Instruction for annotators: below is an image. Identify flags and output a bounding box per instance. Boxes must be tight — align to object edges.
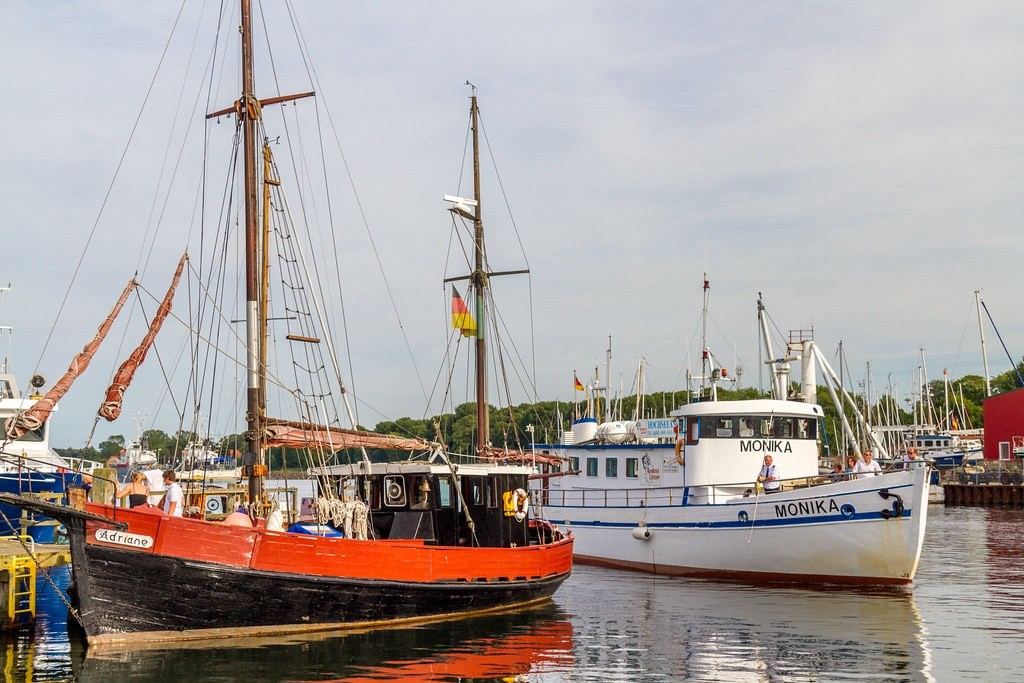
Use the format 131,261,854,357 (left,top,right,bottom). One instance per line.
452,285,477,337
575,375,584,391
951,416,957,430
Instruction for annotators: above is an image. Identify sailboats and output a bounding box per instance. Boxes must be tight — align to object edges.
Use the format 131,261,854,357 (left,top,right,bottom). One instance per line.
818,340,984,485
59,0,575,649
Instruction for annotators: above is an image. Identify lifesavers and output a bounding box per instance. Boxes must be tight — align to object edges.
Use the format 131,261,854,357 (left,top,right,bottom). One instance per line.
513,488,529,519
1010,473,1023,485
502,492,516,516
1001,473,1011,485
675,439,684,465
210,464,218,471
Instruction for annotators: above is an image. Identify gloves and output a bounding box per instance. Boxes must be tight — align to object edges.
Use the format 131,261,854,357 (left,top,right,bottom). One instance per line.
756,485,761,495
757,476,764,483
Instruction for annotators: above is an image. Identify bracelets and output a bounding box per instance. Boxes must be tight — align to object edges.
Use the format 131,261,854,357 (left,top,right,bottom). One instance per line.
116,489,120,490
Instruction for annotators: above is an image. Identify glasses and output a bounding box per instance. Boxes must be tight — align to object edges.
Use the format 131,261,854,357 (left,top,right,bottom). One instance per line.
908,452,915,455
833,467,836,469
863,454,868,456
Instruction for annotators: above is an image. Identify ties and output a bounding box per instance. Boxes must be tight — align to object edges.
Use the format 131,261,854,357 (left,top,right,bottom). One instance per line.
766,468,769,478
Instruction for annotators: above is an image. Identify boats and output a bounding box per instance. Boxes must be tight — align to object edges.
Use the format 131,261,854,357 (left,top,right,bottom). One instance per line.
104,440,200,494
162,440,245,484
0,283,103,546
515,272,934,587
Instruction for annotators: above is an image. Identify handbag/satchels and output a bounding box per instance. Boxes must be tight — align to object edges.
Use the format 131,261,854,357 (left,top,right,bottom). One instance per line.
157,495,165,510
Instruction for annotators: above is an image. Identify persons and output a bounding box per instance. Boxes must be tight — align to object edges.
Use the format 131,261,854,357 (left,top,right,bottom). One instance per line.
817,450,883,484
115,471,150,508
79,472,92,502
755,456,780,496
162,470,185,517
904,448,926,470
